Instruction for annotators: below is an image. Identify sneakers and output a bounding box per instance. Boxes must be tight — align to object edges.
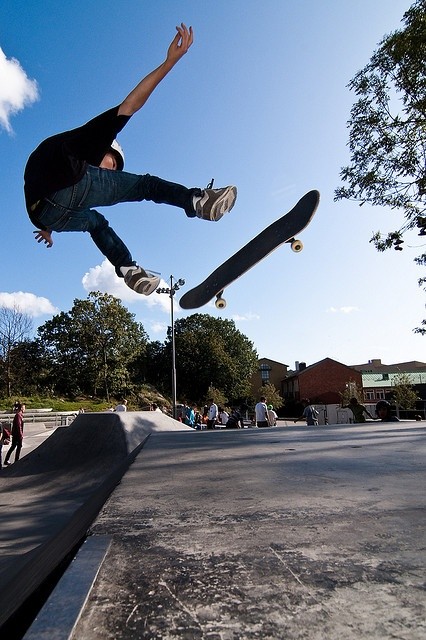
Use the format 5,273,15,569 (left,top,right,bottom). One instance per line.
195,178,237,221
123,267,161,295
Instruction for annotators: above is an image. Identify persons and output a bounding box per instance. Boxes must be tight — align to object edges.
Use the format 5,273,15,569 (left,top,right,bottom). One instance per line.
25,23,237,295
341,398,379,423
375,401,400,422
152,398,244,430
293,398,320,426
114,399,127,412
254,397,270,428
267,404,278,427
0,418,4,470
414,414,422,421
4,402,25,465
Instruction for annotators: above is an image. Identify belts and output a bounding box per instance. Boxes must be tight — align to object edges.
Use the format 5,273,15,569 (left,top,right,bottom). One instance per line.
31,195,50,216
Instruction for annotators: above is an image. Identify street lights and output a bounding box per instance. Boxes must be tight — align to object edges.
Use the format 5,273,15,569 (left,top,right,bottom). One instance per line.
156,275,185,419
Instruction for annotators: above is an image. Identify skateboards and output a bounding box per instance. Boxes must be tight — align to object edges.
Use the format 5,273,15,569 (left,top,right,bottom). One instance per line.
179,190,320,309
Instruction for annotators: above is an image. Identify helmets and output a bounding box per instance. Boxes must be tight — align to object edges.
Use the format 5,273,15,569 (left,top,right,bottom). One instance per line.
376,400,391,410
109,138,124,171
